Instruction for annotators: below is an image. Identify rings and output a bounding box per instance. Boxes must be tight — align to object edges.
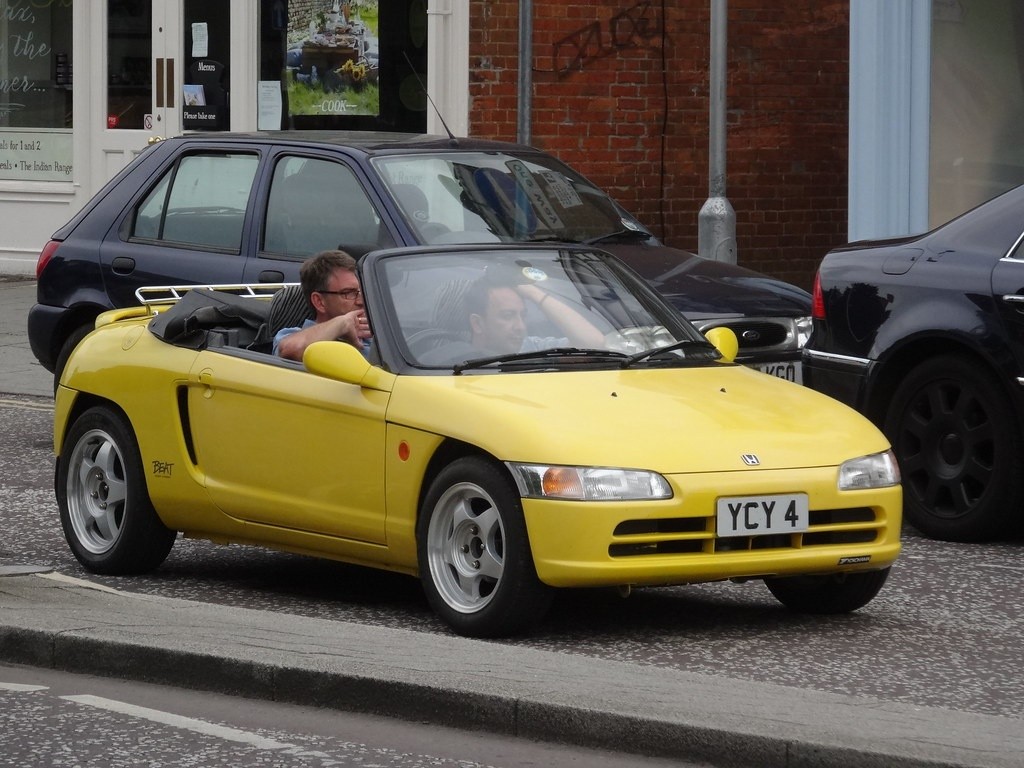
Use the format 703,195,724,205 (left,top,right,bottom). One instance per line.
359,317,362,323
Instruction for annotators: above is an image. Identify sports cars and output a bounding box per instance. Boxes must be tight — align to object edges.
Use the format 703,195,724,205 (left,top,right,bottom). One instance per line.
50,240,904,638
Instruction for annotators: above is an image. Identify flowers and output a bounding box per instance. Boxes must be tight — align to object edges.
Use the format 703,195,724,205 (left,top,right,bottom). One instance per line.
333,58,365,81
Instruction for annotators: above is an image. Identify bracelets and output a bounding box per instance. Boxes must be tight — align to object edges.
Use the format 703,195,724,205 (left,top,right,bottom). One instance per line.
538,293,549,308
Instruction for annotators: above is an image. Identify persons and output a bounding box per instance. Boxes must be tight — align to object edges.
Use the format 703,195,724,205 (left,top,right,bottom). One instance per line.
272,251,374,367
440,275,607,366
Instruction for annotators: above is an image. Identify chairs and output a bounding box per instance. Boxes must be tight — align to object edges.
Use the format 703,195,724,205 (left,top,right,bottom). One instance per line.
429,274,474,346
281,168,450,255
266,285,313,337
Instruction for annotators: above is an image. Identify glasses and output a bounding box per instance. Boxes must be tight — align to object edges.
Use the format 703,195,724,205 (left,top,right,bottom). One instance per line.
319,290,362,300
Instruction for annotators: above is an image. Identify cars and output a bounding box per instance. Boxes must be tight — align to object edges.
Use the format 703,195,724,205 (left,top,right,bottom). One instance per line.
808,180,1024,542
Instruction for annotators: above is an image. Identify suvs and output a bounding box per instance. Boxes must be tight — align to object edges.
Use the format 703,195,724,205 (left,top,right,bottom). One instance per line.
24,125,815,395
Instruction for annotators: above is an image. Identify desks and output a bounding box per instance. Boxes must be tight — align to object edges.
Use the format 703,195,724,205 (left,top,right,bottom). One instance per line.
302,43,364,74
